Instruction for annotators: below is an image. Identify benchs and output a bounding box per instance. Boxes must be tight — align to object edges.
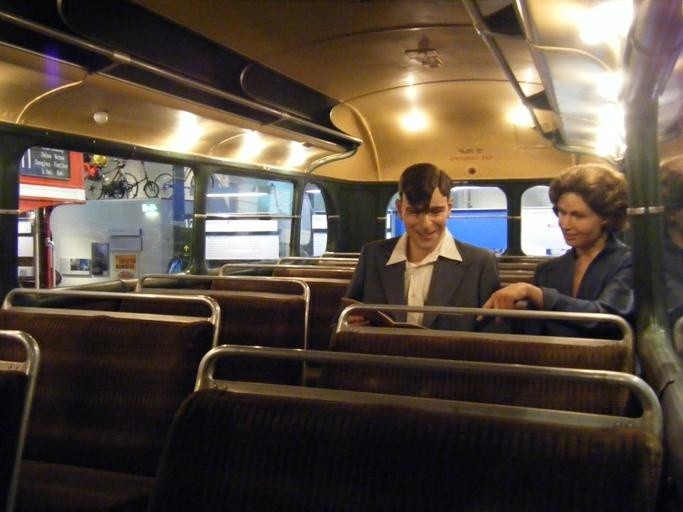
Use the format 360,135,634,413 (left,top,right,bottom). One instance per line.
1,252,666,511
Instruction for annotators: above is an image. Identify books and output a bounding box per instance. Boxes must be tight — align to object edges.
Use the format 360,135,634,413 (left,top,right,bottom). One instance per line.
339,296,431,331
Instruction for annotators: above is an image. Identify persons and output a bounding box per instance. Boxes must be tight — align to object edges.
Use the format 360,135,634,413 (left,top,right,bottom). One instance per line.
329,163,500,338
477,163,637,340
657,166,683,332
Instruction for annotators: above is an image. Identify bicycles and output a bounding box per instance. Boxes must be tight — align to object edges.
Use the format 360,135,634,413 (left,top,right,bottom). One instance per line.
85,155,215,200
